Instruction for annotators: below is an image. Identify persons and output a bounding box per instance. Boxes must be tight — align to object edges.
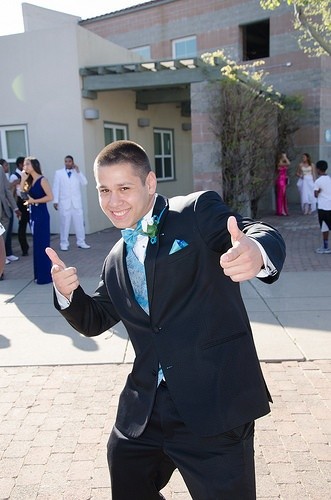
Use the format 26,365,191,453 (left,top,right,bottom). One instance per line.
10,156,36,256
46,141,286,500
296,153,317,215
276,148,290,216
0,158,21,281
312,160,331,254
52,155,92,250
20,156,54,285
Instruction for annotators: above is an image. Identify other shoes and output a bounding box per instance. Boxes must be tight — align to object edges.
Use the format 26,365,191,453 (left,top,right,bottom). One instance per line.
6,255,18,261
22,251,29,256
61,246,68,251
77,243,90,249
5,260,10,264
316,247,331,253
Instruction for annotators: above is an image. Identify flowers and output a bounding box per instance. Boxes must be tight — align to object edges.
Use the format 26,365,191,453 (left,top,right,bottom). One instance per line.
140,215,158,238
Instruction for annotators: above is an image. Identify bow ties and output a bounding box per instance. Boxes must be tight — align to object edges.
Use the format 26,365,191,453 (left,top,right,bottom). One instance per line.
67,169,73,173
121,218,150,249
